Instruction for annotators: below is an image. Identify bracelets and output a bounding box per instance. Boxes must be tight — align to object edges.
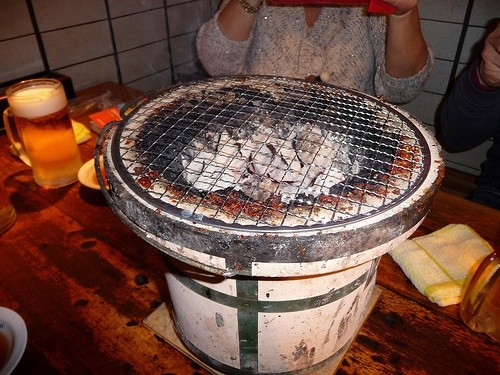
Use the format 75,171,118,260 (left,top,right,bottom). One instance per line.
238,0,260,14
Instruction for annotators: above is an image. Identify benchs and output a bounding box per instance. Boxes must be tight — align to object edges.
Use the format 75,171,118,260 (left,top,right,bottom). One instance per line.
439,159,479,199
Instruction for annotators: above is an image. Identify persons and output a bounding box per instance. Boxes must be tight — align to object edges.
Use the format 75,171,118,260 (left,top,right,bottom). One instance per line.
197,0,435,106
432,22,500,209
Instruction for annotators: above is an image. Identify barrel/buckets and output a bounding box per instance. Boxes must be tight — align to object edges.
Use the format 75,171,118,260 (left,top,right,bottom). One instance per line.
95,75,445,375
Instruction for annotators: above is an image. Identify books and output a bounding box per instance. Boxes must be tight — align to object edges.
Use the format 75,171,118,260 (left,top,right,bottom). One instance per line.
88,96,147,139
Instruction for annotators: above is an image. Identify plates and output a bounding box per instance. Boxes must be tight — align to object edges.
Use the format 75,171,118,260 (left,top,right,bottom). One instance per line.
0,305,27,375
77,153,109,191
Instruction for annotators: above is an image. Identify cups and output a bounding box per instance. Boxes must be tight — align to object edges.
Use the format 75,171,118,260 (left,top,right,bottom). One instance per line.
4,78,82,188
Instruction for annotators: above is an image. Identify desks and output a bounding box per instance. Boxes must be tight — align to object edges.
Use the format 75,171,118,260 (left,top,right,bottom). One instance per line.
0,80,500,375
